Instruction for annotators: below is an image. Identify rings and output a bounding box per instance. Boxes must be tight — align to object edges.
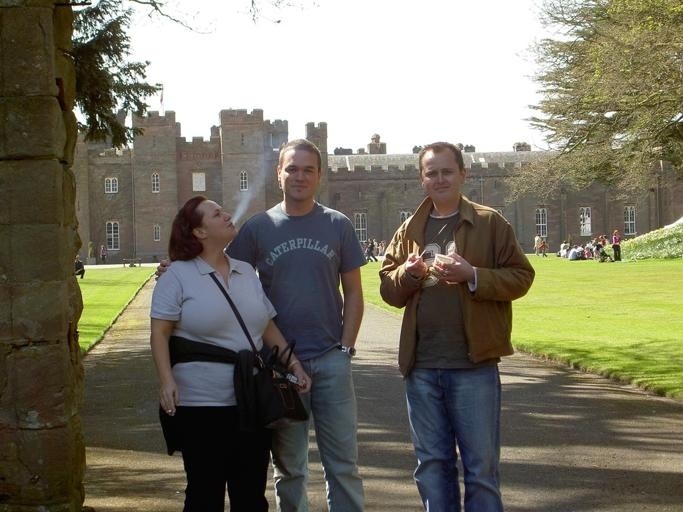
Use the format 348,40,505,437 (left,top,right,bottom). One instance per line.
443,271,449,277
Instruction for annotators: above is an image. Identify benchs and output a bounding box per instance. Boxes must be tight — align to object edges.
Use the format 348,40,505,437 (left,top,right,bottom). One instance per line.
122,258,140,267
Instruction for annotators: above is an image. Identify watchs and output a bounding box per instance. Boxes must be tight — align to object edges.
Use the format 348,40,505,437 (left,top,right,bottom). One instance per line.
339,346,358,356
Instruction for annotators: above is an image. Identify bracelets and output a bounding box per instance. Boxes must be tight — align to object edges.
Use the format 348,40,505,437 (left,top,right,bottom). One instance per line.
287,359,300,372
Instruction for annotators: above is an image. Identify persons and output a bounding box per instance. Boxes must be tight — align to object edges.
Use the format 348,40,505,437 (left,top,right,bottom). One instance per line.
376,139,537,510
99,246,107,265
148,193,313,512
154,136,370,511
360,237,387,264
73,255,86,279
532,228,624,264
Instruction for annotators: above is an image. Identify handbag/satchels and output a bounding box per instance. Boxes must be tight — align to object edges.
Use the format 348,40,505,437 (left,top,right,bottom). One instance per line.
234,349,298,427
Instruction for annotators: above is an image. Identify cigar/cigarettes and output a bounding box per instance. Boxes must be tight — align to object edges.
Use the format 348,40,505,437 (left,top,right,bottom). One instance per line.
166,409,172,413
